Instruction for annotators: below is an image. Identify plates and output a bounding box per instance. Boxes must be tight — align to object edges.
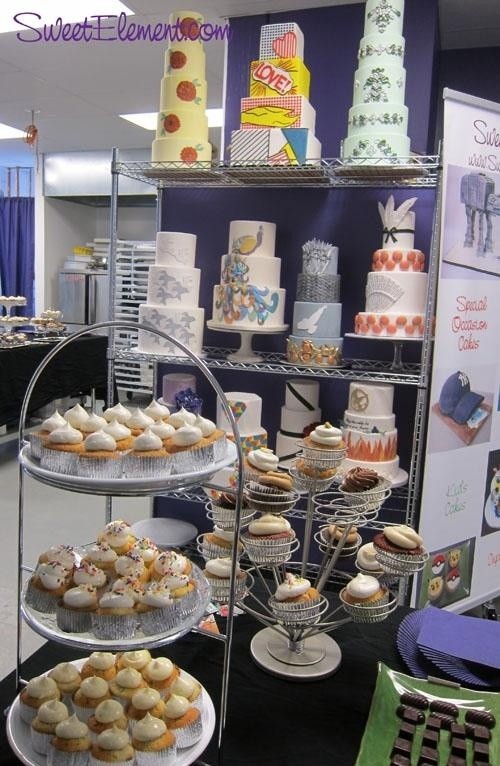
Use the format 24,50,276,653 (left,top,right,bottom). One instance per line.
34,326,64,331
484,494,500,531
34,336,68,343
349,660,499,765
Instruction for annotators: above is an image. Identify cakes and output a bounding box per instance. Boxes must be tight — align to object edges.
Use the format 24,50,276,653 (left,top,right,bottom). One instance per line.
343,0,411,165
355,195,429,338
0,296,67,341
137,230,205,357
231,23,323,167
213,219,287,327
152,10,212,169
286,237,345,369
428,550,461,601
489,467,500,517
19,373,424,766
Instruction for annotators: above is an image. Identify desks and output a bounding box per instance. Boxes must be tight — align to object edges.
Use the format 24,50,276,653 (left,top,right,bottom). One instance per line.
0,552,500,766
0,325,108,435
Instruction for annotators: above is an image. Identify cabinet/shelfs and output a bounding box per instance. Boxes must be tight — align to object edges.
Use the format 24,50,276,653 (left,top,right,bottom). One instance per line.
105,138,444,606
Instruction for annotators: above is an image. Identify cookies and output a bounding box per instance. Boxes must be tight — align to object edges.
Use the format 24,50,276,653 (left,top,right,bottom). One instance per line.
389,693,496,766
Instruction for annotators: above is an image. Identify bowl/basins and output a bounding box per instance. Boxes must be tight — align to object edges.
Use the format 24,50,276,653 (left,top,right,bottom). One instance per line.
89,255,107,266
